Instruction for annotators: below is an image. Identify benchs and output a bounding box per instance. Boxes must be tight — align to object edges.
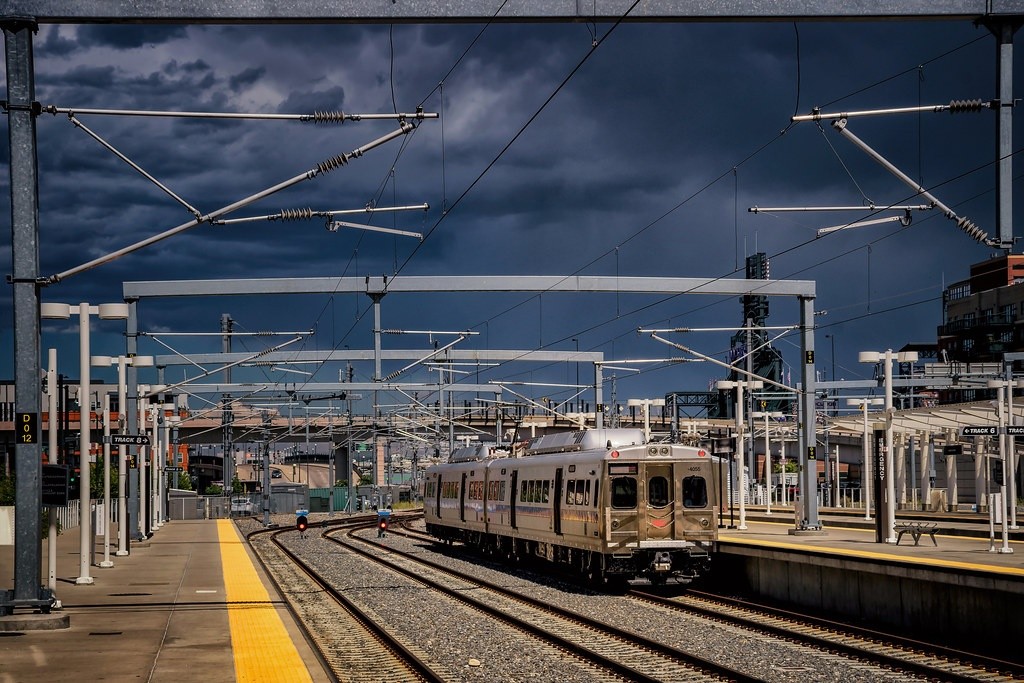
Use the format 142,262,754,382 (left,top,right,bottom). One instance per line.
894,521,941,546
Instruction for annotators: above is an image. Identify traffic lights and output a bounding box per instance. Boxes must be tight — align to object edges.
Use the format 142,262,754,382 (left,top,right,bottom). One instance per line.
377,516,388,532
296,516,308,531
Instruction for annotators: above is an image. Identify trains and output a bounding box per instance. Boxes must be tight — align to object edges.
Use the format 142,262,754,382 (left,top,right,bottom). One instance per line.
423,428,721,596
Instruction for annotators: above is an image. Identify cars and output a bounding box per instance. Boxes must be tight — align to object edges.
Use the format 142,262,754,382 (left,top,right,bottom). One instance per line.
230,497,258,517
360,489,393,513
271,469,282,479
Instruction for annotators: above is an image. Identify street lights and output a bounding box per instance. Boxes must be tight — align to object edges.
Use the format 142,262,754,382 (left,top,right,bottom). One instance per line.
147,415,180,528
987,379,1024,530
125,385,168,540
716,380,764,529
91,356,154,556
826,334,835,396
39,303,127,586
858,350,918,543
137,402,176,530
572,339,579,413
628,398,666,442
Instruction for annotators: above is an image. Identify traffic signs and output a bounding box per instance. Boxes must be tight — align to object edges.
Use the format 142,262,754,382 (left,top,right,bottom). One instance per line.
111,434,152,447
